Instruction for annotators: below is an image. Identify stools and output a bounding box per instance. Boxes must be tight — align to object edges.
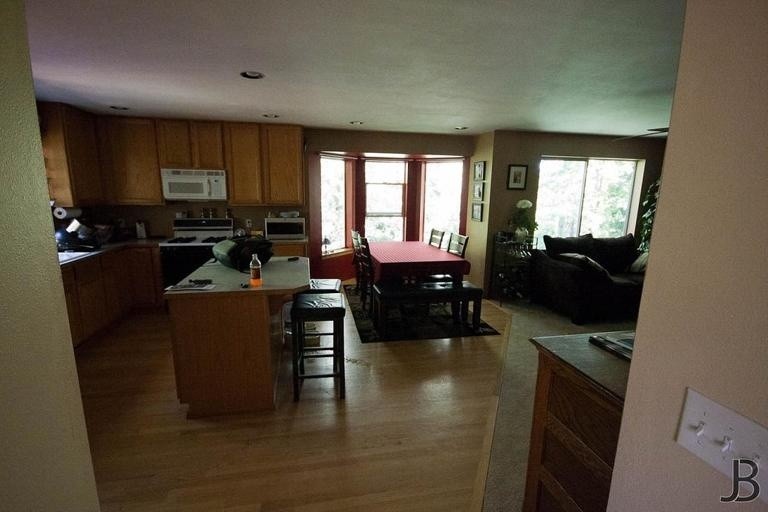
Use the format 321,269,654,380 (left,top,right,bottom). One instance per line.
291,293,346,402
293,278,341,352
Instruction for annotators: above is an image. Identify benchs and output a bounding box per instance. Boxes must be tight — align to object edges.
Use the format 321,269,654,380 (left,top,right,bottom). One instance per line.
373,281,482,330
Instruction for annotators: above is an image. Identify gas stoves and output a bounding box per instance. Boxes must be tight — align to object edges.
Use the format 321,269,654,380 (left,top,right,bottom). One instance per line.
158,236,229,245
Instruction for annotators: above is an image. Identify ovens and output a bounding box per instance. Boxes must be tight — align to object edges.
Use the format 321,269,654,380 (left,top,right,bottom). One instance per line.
160,246,216,303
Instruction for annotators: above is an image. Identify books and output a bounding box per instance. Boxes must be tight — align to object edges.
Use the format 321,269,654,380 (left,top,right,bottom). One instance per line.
589,333,636,362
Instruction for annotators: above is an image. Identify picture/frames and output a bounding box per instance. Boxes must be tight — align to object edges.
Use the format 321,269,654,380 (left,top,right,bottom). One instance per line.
471,203,483,222
474,161,485,181
507,164,528,190
472,183,484,201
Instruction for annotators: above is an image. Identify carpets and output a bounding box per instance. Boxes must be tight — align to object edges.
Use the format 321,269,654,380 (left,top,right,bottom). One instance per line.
471,300,636,512
342,277,501,343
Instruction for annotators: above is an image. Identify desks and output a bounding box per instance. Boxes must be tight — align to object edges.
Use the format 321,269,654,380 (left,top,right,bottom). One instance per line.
522,331,633,512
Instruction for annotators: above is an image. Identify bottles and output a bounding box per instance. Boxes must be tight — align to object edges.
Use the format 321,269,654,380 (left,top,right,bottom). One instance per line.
249,253,261,288
200,207,229,218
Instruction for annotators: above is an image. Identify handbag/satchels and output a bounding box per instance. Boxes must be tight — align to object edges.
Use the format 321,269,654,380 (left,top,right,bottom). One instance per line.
212,235,274,272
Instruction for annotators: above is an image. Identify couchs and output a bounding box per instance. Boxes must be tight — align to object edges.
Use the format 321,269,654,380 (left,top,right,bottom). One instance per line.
534,250,646,323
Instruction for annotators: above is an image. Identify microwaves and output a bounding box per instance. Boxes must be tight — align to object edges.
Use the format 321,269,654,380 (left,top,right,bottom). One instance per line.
160,168,229,202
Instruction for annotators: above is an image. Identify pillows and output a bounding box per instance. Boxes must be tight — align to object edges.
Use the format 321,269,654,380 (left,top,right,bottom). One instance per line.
626,252,649,273
542,234,594,260
593,232,636,273
560,253,613,282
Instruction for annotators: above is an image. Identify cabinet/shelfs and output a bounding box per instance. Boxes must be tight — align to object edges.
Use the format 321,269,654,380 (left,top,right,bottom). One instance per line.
61,246,165,348
272,245,305,257
96,116,172,206
223,122,306,207
491,232,538,307
154,118,223,170
36,102,104,208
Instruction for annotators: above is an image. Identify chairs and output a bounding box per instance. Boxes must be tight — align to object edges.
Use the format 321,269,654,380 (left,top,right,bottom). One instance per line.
351,230,372,312
408,229,469,315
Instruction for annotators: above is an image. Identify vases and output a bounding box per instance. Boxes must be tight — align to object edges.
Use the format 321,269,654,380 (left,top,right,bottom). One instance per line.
516,227,529,241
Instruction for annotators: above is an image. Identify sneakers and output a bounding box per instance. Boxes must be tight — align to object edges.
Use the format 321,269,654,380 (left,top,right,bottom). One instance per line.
283,323,320,347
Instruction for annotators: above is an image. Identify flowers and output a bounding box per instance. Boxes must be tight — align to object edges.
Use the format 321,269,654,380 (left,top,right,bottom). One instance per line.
508,200,539,231
